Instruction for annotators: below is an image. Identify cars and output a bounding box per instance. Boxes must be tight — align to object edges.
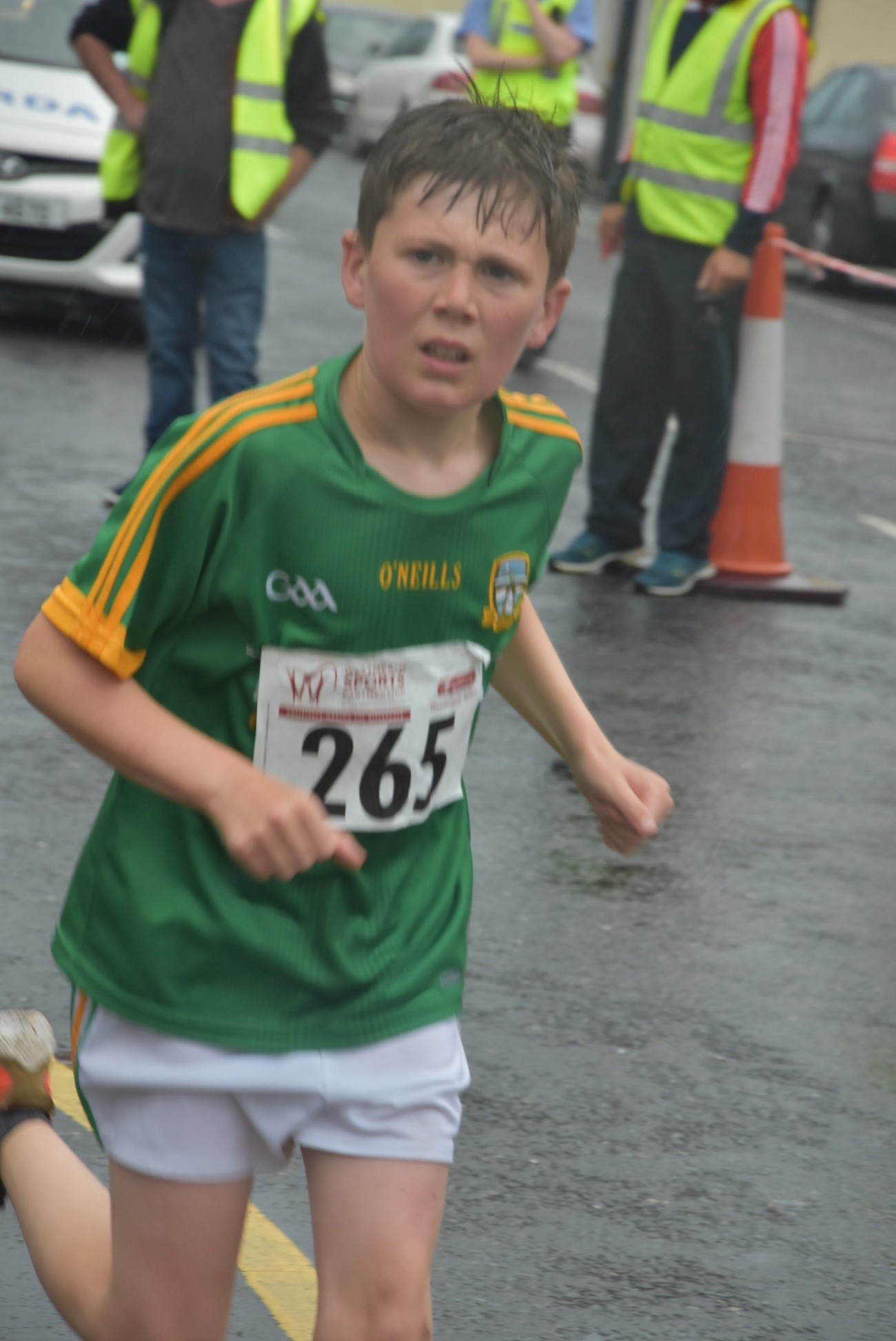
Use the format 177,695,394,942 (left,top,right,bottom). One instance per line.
344,16,609,174
0,0,210,339
763,64,895,292
316,2,388,116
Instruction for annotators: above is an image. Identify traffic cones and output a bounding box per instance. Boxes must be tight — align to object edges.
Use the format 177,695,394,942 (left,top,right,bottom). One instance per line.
604,221,856,607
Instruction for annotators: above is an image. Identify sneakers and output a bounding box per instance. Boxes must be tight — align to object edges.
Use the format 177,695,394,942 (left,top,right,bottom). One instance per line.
636,548,718,598
547,527,647,574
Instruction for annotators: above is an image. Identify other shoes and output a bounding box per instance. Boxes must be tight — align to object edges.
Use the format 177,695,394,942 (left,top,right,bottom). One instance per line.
103,482,130,505
1,1007,58,1112
516,351,535,367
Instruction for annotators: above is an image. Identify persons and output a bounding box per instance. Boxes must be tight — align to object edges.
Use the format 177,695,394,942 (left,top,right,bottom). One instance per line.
546,1,809,601
460,2,600,369
0,100,669,1339
68,0,335,503
